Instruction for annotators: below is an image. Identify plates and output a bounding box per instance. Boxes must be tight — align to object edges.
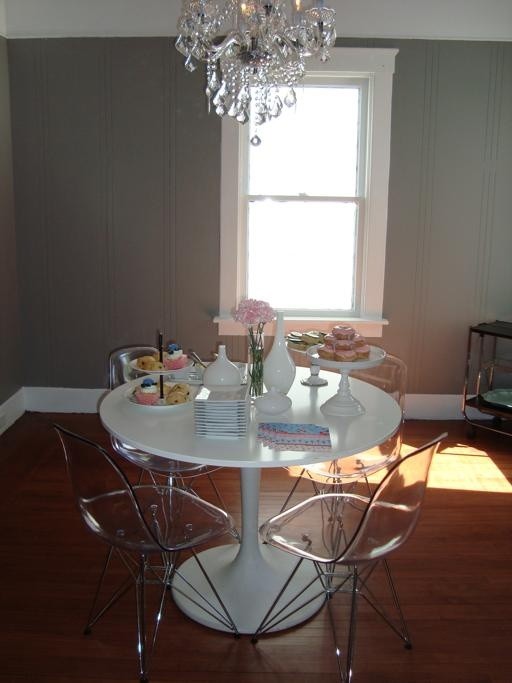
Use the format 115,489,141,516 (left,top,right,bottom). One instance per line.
192,383,252,441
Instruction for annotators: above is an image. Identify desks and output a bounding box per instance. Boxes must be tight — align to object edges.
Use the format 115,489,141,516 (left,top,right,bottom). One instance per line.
99,361,403,637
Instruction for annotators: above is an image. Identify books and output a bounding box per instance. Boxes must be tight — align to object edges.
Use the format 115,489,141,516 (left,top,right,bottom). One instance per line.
194,384,252,439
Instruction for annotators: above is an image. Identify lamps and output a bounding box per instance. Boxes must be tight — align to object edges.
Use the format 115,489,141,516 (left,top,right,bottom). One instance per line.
174,1,337,148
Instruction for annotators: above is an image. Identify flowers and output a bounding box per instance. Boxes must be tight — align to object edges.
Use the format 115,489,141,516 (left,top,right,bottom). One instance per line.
231,298,276,397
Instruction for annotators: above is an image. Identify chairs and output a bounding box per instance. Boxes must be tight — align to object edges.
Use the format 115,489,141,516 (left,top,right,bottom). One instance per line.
54,425,241,683
251,431,447,683
108,345,241,540
277,355,409,516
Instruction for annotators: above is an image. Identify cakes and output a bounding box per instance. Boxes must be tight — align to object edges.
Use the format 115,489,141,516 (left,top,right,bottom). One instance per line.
134,343,192,405
287,325,371,362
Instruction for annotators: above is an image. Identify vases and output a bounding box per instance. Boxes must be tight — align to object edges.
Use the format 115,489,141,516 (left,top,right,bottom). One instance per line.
245,324,264,400
